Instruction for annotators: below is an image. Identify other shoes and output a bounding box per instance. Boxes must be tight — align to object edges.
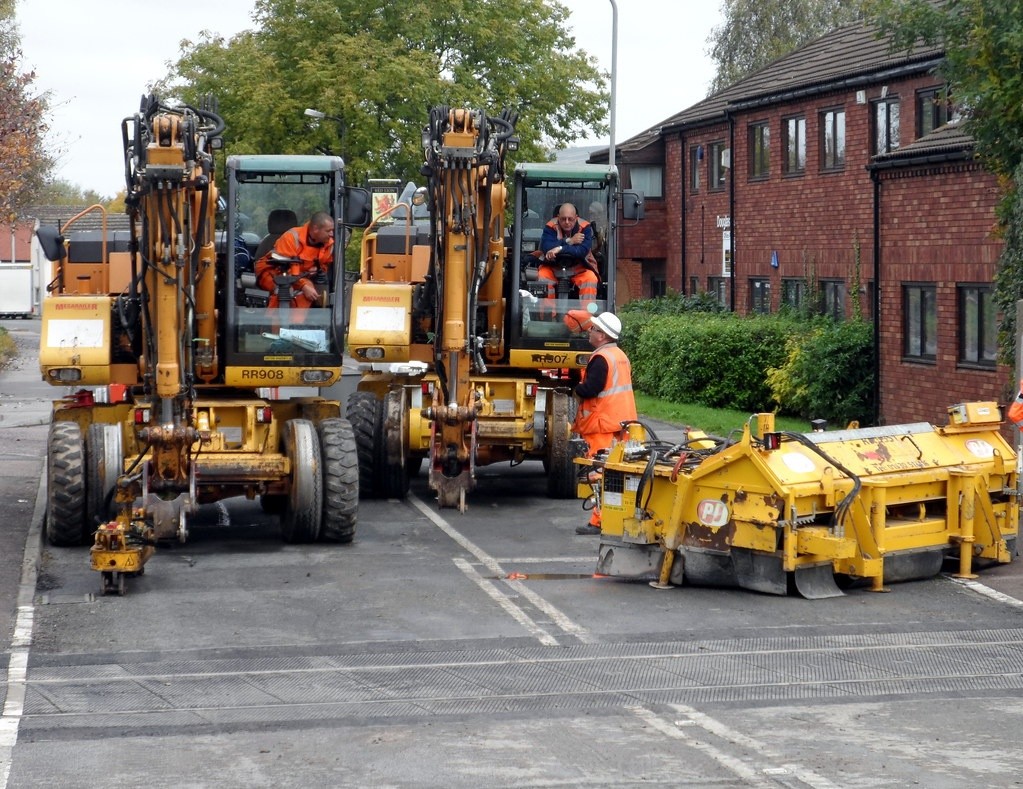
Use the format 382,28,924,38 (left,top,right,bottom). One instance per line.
575,524,602,535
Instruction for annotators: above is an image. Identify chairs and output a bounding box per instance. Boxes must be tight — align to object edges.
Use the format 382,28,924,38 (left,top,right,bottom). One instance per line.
257,207,302,263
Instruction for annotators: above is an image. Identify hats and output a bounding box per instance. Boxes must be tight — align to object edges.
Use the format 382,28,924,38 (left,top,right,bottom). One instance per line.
590,311,622,341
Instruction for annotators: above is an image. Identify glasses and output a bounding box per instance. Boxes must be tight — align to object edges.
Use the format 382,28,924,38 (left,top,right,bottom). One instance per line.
558,216,577,222
591,325,604,334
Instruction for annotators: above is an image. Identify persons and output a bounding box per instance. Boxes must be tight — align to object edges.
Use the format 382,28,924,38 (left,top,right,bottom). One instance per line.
566,312,642,535
535,204,597,318
254,212,336,337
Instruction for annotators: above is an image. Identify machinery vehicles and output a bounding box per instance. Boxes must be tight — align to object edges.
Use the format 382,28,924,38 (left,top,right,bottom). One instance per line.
346,93,626,507
32,85,363,599
